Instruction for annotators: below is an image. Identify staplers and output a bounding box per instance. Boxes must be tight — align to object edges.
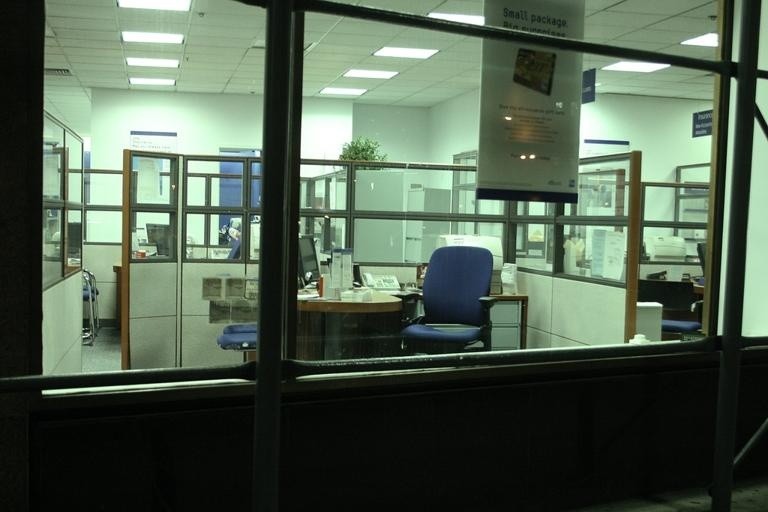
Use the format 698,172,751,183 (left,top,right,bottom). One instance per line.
646,270,666,281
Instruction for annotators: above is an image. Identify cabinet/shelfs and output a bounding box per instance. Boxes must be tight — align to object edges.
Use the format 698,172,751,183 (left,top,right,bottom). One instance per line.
415,295,529,351
638,279,694,319
636,301,664,341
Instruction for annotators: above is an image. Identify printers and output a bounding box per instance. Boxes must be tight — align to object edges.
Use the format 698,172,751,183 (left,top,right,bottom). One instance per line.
644,235,687,262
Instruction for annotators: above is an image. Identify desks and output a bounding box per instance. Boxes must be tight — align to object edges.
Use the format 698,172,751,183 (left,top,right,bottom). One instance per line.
298,288,403,361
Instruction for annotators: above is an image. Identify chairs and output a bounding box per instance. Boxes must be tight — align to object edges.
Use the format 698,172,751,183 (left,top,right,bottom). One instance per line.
662,319,701,341
393,246,495,355
216,323,258,350
83,268,102,345
227,243,238,258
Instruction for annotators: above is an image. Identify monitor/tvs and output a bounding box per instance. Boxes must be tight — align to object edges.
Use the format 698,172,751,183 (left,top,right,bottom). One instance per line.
146,223,175,255
293,237,321,285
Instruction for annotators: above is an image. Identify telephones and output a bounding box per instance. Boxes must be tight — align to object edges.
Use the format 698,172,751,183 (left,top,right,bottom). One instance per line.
361,272,401,291
